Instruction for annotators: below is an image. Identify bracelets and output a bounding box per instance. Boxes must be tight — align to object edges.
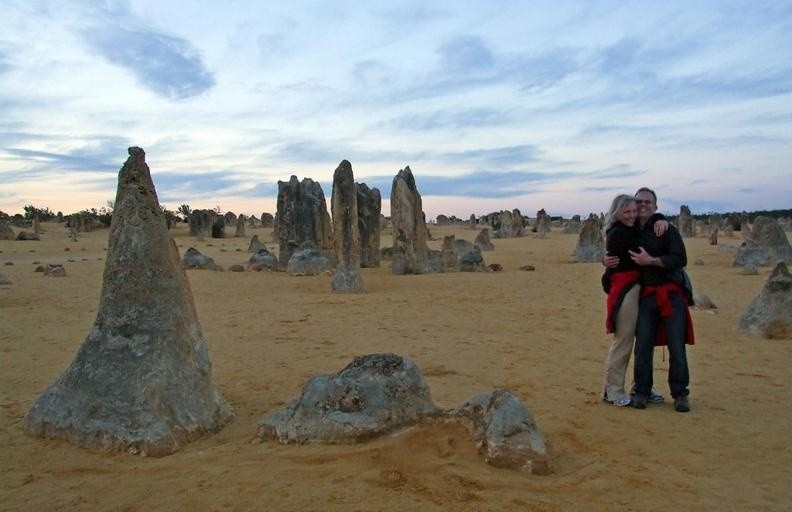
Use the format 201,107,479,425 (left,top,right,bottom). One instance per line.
649,257,656,265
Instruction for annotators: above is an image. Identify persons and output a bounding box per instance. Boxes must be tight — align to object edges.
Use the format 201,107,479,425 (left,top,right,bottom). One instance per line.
599,187,692,412
596,194,668,406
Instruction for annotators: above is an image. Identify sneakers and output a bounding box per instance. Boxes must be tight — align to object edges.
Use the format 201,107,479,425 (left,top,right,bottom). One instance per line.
631,386,664,403
604,392,631,406
633,391,648,408
674,396,690,411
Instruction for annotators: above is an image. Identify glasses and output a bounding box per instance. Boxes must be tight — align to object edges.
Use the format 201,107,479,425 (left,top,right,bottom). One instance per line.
636,198,651,204
